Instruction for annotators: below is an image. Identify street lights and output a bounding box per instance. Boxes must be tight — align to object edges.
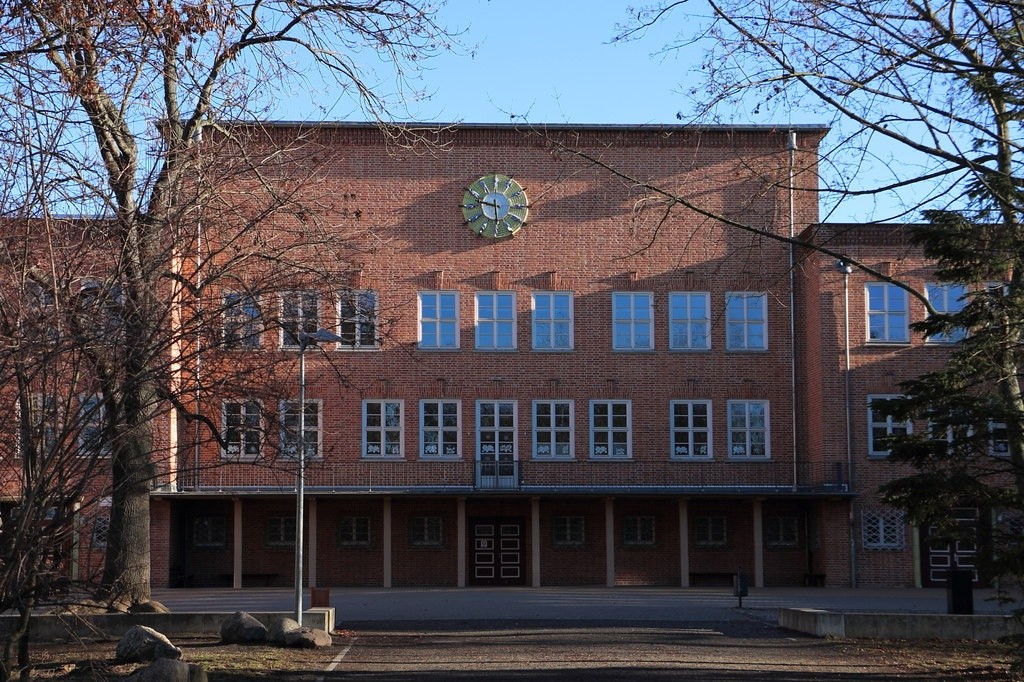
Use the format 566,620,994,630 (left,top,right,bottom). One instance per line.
295,329,354,625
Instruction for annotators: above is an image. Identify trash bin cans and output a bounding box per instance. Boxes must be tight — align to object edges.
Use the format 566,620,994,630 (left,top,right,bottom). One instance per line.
946,568,973,615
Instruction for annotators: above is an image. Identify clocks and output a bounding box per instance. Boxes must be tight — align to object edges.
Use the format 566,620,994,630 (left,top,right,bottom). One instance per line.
457,172,532,241
